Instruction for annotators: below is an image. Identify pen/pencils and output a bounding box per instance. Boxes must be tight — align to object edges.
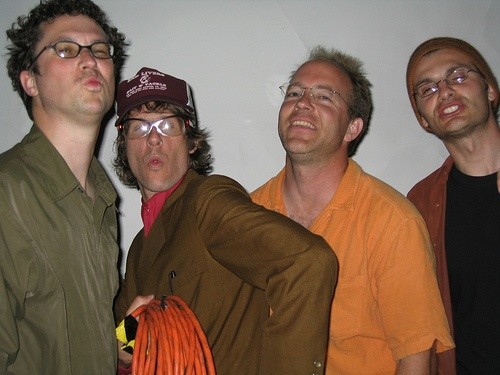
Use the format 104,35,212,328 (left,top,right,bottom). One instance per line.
168,269,177,294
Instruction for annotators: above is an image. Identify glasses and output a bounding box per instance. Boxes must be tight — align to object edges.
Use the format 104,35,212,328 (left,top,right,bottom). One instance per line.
277,80,356,112
30,41,117,68
407,67,490,100
112,115,190,138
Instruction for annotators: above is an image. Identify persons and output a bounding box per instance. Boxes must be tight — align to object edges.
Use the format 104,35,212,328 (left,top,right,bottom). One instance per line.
0,0,130,375
112,67,338,375
406,37,500,375
248,44,456,375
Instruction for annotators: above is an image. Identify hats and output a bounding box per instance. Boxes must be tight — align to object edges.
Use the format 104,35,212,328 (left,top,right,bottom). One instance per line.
114,67,194,128
